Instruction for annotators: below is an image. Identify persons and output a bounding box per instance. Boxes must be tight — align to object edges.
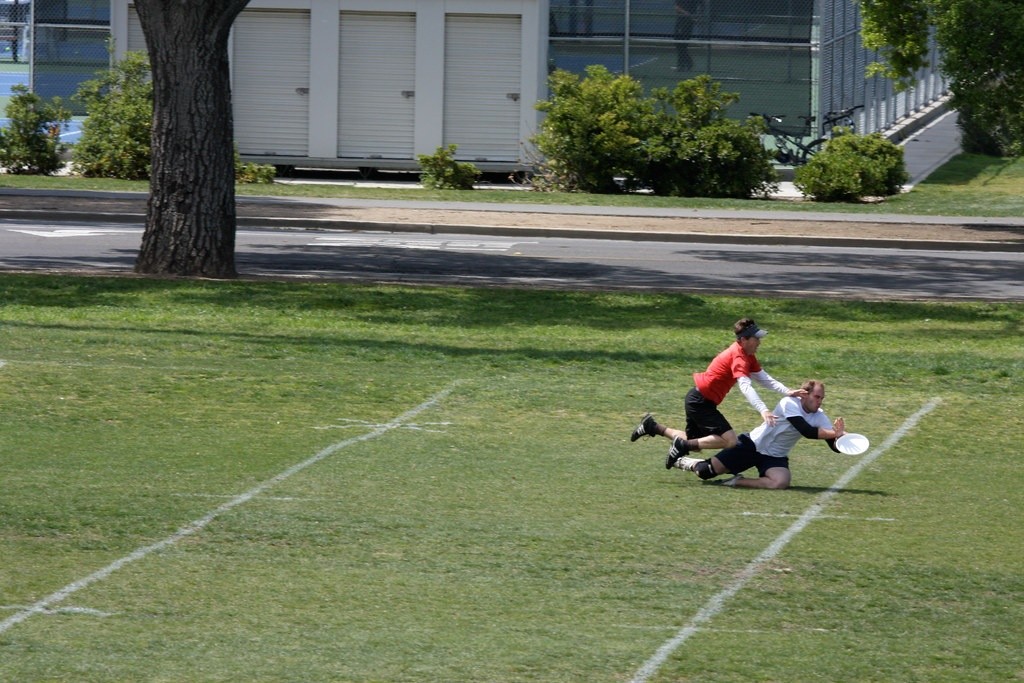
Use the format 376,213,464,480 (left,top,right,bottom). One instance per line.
631,317,808,469
672,378,851,491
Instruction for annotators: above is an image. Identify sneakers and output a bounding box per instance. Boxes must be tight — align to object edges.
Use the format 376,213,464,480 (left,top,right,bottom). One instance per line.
674,457,705,471
631,414,657,442
720,474,744,487
666,436,689,470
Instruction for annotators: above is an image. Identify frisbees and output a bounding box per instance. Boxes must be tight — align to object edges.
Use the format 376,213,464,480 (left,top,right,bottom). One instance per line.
835,432,870,456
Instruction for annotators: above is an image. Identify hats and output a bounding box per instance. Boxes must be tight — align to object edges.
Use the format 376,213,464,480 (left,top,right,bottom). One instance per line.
736,324,768,338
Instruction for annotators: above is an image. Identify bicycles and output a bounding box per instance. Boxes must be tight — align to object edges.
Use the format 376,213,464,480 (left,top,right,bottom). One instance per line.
747,102,864,166
